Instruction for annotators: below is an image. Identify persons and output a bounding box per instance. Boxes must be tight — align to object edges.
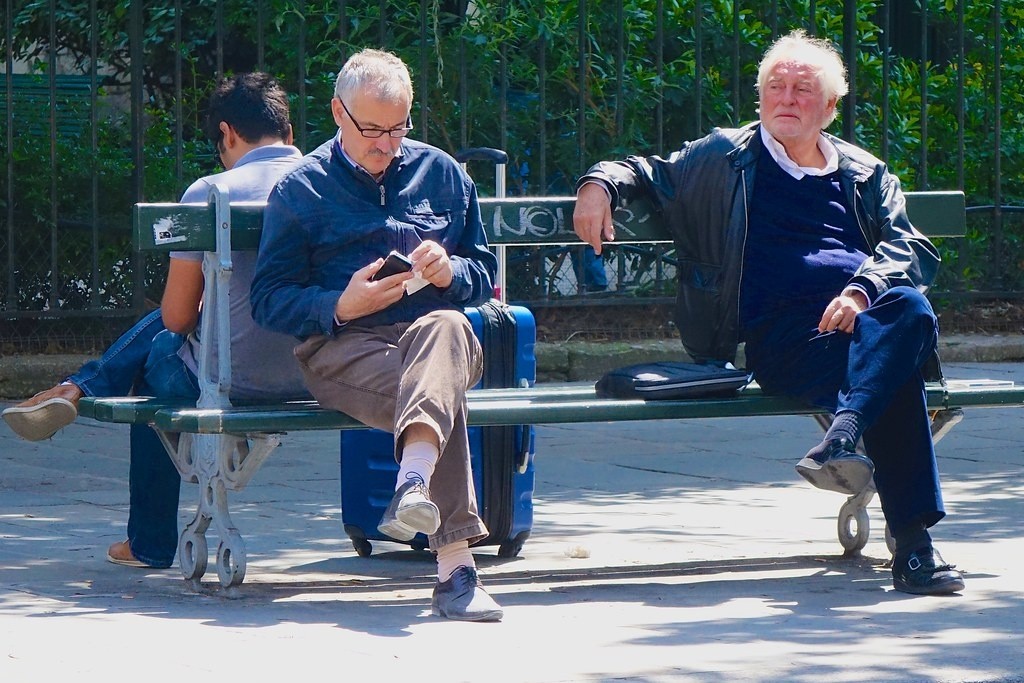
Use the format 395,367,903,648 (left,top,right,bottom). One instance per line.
248,49,504,621
1,71,302,567
572,32,965,594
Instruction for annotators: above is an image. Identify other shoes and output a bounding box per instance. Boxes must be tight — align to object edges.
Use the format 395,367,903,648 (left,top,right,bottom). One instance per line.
106,538,150,567
2,383,84,442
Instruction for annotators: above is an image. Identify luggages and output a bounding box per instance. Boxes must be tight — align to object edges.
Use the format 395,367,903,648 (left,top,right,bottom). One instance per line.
340,148,538,560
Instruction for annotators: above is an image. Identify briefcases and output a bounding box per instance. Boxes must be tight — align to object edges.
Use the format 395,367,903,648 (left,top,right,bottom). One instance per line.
594,362,753,400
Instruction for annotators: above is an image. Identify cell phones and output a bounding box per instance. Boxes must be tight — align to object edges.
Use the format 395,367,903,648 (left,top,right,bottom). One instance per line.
372,250,414,281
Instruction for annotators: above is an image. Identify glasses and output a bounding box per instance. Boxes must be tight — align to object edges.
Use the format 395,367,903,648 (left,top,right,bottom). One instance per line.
214,130,223,165
338,95,413,138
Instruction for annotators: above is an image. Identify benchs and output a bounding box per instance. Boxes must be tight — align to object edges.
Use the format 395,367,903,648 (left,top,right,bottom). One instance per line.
75,186,1023,598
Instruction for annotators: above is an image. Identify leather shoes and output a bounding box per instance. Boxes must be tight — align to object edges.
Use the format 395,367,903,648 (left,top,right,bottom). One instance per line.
377,471,441,542
431,565,503,621
794,445,874,494
892,548,965,594
